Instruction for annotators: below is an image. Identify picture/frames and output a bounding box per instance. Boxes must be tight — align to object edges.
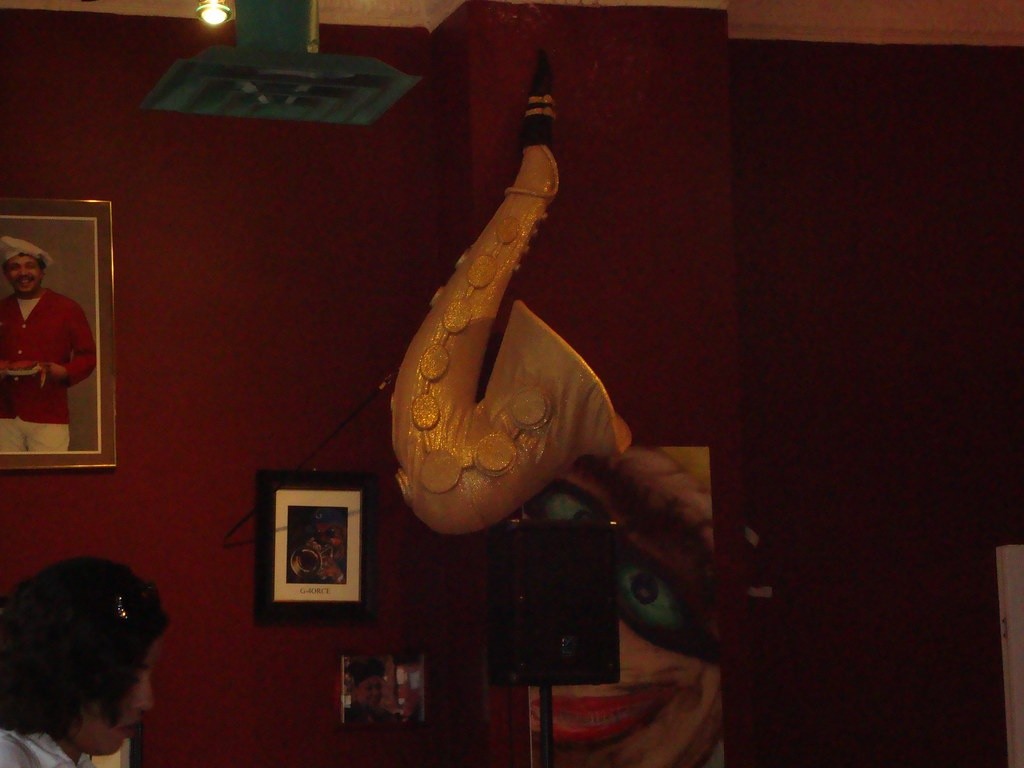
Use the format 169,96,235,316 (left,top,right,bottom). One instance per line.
335,649,434,732
513,446,726,768
253,469,375,623
2,198,116,469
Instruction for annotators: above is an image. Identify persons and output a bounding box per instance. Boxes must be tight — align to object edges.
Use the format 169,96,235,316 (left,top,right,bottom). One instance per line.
1,554,170,768
343,659,405,722
2,235,97,452
292,508,345,583
